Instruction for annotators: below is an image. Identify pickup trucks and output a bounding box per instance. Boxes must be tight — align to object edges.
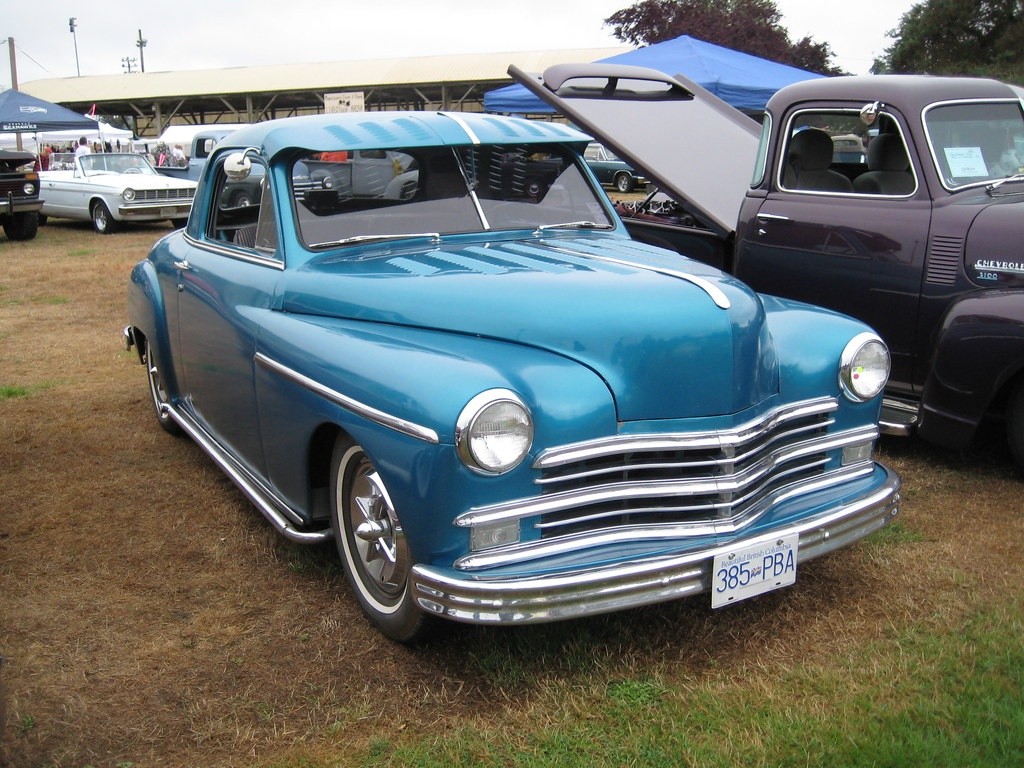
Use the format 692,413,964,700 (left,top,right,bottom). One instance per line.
489,60,1024,480
510,138,652,200
152,129,335,219
301,146,425,201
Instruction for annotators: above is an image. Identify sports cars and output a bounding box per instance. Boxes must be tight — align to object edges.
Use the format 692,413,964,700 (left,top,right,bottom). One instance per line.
38,150,202,235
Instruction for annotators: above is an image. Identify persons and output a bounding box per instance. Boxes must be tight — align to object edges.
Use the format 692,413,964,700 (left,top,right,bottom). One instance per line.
44,141,74,163
171,144,185,163
32,143,48,173
74,136,93,171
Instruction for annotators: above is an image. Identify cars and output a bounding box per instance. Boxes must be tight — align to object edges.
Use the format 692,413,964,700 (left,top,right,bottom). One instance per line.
123,108,902,650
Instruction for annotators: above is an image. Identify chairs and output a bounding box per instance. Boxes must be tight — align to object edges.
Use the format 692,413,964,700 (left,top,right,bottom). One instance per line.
784,127,915,196
